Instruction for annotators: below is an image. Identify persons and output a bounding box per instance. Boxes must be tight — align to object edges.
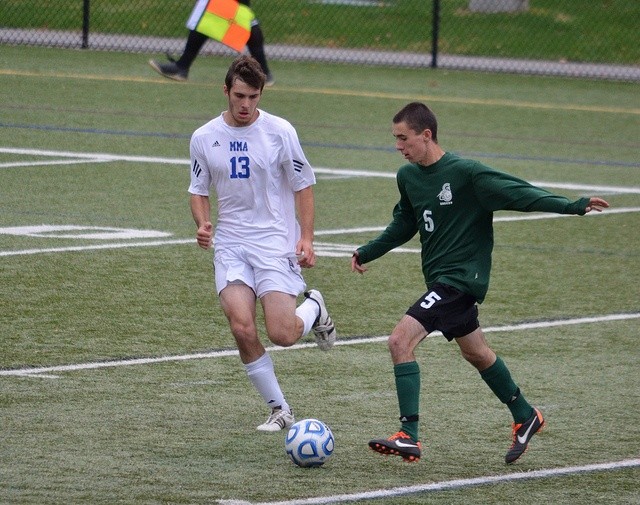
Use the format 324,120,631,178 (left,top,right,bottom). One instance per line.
349,101,610,464
147,0,277,89
187,55,338,435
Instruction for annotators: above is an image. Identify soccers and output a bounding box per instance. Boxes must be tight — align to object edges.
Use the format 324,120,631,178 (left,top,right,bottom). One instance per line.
284,419,335,468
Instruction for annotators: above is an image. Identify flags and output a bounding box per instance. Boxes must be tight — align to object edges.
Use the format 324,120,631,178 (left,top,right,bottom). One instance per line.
185,0,256,53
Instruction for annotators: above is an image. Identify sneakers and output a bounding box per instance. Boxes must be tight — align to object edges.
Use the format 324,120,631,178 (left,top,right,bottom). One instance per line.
304,289,336,352
149,60,189,82
256,410,296,431
264,73,274,86
505,407,545,464
368,431,422,462
